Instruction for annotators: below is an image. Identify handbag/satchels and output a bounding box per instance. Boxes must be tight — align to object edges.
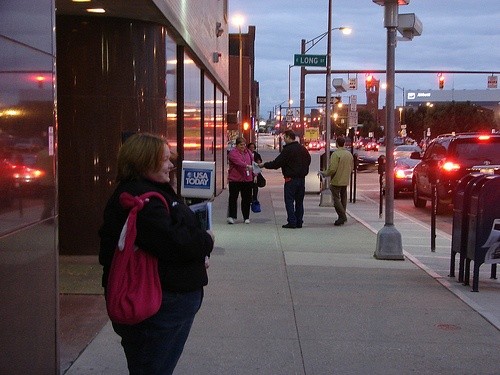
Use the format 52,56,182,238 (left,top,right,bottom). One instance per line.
252,201,261,213
252,161,261,175
106,192,170,325
257,173,266,187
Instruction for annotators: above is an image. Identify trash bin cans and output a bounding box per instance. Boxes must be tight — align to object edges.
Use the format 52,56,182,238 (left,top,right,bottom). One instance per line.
320,149,337,189
446,172,500,293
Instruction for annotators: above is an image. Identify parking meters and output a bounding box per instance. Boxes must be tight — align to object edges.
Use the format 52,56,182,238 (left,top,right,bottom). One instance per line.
427,153,442,252
376,154,385,218
353,153,360,202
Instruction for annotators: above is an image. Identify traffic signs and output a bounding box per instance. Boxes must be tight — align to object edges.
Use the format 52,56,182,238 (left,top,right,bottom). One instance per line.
317,95,341,106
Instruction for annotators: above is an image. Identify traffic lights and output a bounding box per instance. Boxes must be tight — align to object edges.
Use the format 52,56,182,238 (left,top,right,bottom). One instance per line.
438,76,445,89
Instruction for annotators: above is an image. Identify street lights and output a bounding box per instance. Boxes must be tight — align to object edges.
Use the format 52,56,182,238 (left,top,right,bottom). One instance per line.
299,26,352,148
273,100,292,149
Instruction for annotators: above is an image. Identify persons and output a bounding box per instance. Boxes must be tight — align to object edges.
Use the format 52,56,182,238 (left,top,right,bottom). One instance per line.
225,137,257,224
246,143,266,204
258,130,311,229
320,137,354,226
99,133,215,375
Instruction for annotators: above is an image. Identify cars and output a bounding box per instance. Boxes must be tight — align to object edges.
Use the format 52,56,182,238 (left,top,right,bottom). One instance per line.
382,144,425,199
354,138,379,151
411,131,500,217
307,142,325,150
352,148,380,171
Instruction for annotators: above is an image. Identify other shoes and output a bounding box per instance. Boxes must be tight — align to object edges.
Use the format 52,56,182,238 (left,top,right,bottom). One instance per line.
227,217,234,224
334,218,347,225
297,223,302,228
245,219,250,224
282,223,296,228
